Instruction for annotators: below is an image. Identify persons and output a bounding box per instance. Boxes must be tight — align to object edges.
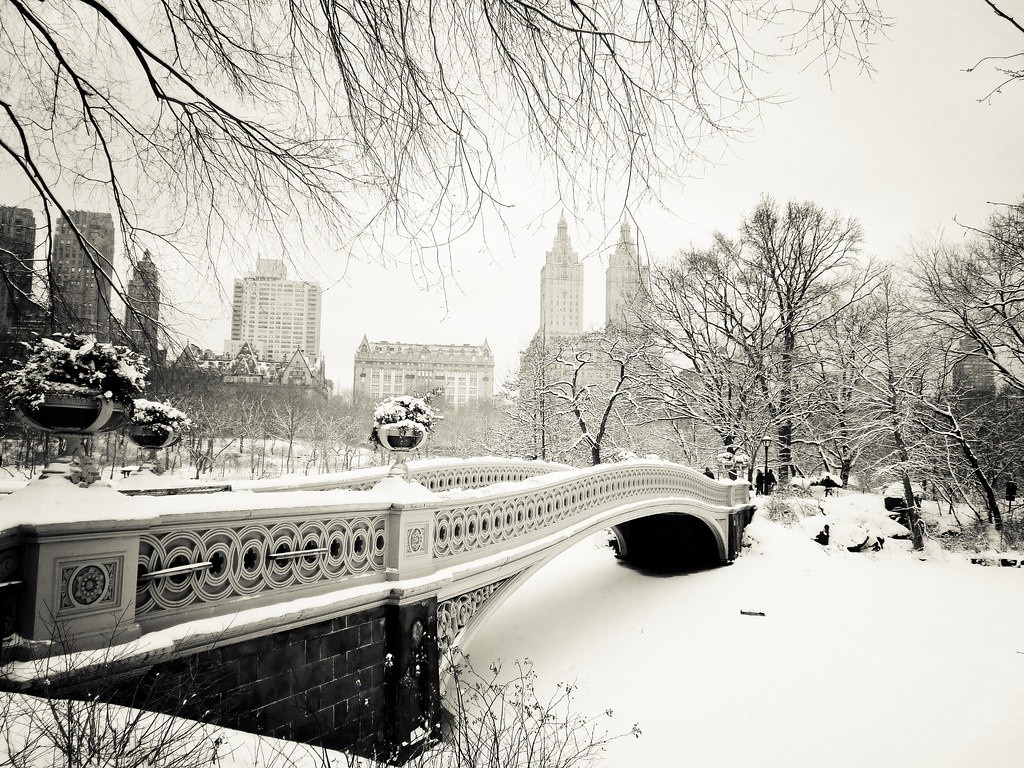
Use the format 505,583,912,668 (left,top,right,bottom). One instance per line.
756,470,764,495
812,525,829,546
824,476,832,497
703,467,715,479
768,469,777,489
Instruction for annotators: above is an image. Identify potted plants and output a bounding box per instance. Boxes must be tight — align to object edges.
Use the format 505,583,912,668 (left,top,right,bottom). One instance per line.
368,394,433,452
125,398,191,449
2,332,153,435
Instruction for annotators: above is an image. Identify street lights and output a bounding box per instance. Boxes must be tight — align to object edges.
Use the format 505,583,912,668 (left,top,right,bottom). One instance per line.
761,435,773,495
